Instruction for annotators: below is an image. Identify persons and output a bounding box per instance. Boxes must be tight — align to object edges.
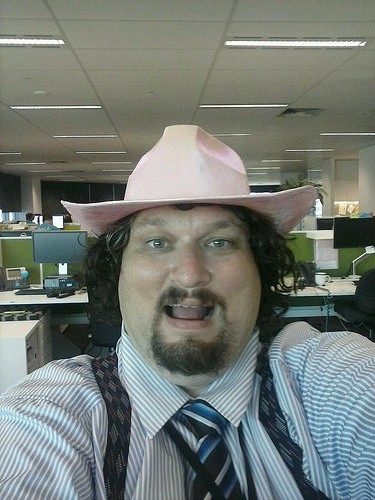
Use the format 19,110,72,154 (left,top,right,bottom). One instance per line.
35,214,60,230
24,213,37,224
1,124,375,500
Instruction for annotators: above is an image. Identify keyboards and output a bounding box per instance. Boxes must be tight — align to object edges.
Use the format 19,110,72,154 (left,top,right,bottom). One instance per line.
15,289,76,295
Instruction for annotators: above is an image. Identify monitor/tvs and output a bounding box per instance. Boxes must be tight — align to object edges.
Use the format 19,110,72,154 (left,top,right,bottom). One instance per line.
51,214,64,229
333,217,375,249
0,230,33,236
315,216,350,231
31,215,44,225
31,230,89,264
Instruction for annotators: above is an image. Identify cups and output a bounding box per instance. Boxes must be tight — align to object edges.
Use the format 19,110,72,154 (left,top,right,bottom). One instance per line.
315,272,331,285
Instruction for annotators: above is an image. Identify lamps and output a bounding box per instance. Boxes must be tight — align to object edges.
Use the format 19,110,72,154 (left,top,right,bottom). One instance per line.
349,246,375,281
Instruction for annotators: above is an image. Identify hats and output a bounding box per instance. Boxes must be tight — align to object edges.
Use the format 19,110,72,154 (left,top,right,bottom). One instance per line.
60,124,316,238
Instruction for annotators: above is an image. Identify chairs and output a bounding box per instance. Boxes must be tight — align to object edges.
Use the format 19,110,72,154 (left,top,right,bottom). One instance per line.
334,269,375,344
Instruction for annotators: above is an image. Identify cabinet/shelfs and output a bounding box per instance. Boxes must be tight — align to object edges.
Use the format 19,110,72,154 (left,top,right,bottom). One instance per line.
0,321,40,392
1,308,52,367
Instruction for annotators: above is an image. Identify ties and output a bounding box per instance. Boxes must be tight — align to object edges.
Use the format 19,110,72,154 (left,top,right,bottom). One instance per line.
171,399,248,500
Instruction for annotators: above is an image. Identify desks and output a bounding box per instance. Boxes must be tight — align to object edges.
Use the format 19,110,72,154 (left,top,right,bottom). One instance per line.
0,275,355,323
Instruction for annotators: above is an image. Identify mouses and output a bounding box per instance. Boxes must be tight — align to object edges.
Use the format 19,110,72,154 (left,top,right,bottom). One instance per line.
47,290,63,298
